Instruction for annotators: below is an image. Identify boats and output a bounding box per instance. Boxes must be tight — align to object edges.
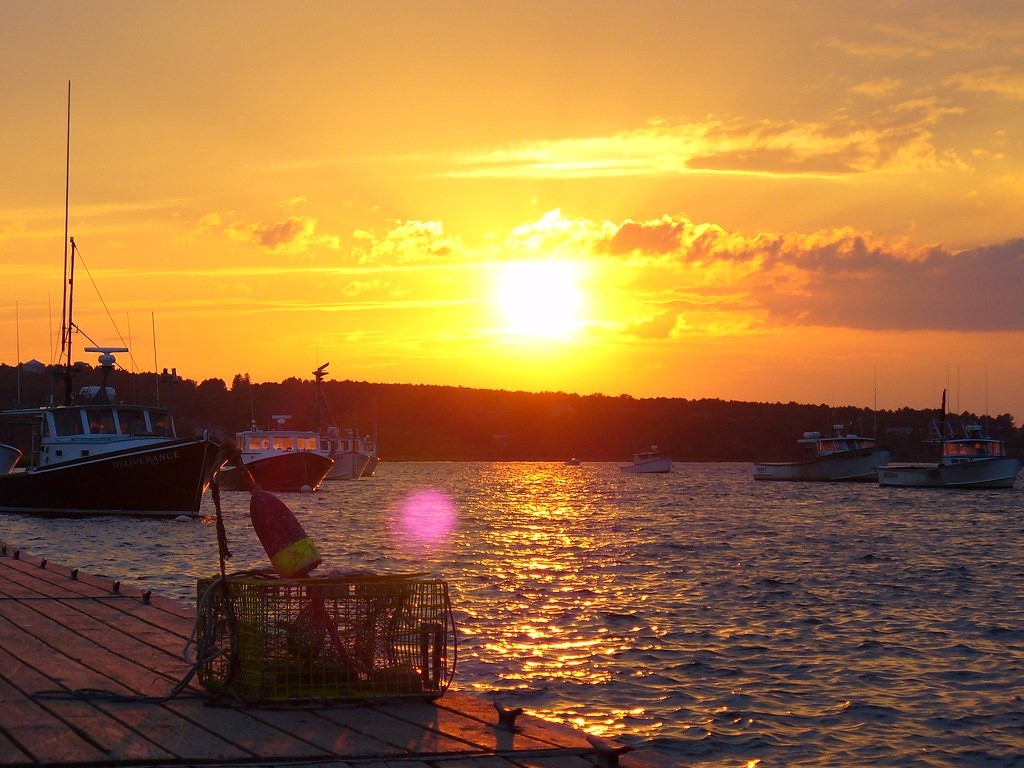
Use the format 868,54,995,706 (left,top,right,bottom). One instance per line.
0,233,236,521
871,388,1024,488
318,425,381,482
619,450,671,475
748,423,893,483
210,384,336,494
